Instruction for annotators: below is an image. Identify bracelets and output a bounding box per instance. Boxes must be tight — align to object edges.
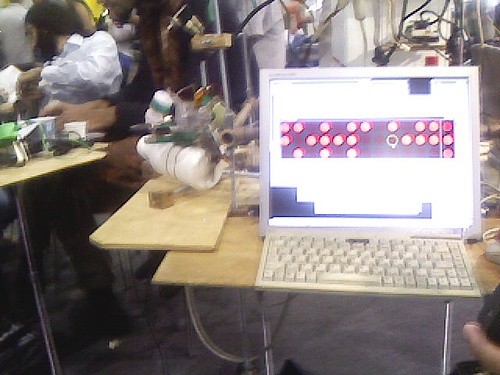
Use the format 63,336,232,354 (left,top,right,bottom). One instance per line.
464,324,479,329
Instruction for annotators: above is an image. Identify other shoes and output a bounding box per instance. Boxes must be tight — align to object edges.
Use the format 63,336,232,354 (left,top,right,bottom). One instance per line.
135,250,164,279
159,285,180,299
19,248,42,278
62,292,117,313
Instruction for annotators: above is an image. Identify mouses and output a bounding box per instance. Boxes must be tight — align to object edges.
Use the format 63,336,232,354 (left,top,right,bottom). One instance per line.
483,243,500,263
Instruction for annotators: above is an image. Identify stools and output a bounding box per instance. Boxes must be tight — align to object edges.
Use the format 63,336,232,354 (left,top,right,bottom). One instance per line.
52,193,196,357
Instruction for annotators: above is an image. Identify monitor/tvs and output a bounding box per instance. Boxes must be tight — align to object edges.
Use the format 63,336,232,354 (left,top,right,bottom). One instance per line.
259,67,483,242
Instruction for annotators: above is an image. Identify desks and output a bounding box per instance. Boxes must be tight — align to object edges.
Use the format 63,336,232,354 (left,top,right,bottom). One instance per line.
0,141,500,375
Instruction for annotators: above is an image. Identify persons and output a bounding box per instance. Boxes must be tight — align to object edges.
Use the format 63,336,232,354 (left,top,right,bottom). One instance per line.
0,0,500,375
13,0,286,292
461,320,500,375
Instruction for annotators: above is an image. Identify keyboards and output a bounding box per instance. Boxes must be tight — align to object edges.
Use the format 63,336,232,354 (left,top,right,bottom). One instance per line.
254,228,481,298
